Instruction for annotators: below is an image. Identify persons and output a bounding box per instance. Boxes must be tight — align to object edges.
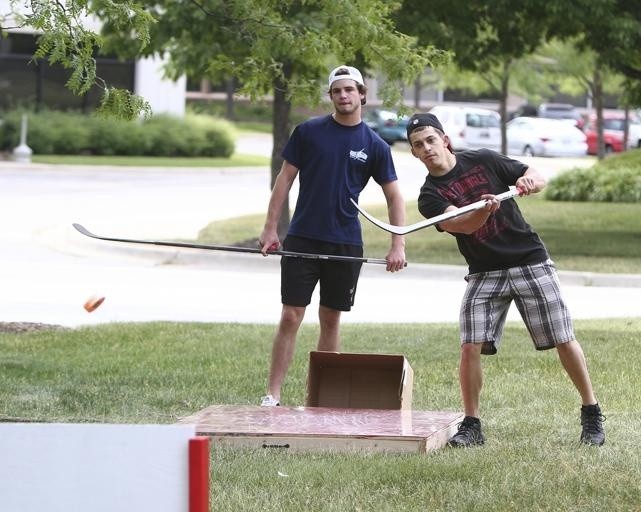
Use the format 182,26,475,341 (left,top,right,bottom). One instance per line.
257,64,408,408
406,112,607,447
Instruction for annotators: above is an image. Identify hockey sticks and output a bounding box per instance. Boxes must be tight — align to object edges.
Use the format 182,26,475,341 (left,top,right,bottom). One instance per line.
73,222,408,265
350,186,525,235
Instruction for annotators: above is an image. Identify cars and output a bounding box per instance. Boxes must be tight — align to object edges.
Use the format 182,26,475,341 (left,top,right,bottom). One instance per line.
361,102,641,157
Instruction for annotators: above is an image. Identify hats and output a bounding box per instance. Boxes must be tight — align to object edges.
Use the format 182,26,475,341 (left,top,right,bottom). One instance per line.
407,113,445,137
329,65,365,91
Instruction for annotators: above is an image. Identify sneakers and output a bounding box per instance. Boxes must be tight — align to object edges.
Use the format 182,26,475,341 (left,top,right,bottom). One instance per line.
260,394,282,407
580,402,606,446
448,416,486,448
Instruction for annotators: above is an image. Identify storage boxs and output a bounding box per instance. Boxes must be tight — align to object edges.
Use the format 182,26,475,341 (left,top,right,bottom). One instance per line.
306,351,414,410
